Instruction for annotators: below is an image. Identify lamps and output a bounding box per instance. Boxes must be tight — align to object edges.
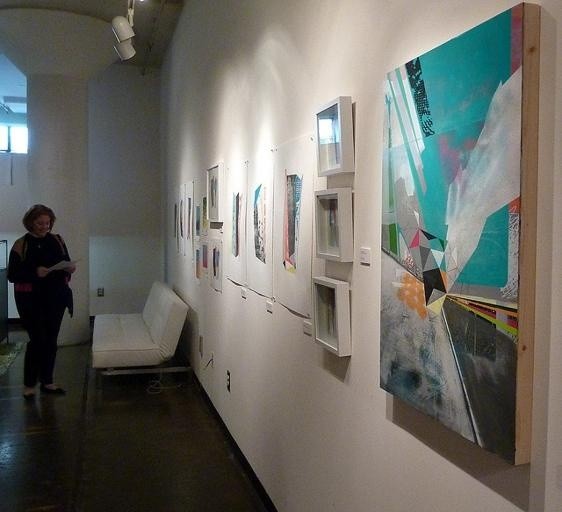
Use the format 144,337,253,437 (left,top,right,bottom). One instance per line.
107,15,137,64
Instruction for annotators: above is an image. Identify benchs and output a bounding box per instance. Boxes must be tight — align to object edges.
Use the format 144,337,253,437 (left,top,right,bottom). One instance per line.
83,279,192,419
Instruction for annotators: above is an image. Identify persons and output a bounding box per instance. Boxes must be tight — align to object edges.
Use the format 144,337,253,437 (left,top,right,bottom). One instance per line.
7,204,79,401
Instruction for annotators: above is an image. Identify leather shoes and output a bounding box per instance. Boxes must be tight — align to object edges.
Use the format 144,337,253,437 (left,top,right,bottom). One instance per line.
40,384,66,395
23,387,36,401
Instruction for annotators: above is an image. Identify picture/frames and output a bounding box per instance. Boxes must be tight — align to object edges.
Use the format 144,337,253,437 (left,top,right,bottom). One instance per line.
204,159,227,226
308,94,356,359
